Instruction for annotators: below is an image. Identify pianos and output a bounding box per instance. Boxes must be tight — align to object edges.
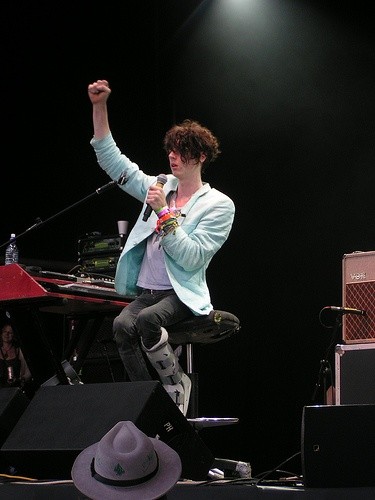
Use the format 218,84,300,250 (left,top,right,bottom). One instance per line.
0,263,131,313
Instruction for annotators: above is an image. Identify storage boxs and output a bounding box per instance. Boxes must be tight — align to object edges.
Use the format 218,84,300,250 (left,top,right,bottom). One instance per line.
335,343,375,404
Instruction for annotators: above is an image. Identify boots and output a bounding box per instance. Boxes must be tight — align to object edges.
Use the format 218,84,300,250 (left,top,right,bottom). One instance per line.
141,327,192,416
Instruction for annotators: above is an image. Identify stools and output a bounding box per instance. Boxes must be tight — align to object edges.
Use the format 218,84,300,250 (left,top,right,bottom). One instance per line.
167,310,252,480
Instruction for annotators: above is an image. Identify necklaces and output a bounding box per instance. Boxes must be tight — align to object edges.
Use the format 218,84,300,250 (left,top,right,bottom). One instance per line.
3,345,14,358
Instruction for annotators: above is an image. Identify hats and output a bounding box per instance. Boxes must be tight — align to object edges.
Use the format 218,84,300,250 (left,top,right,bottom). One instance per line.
70,420,183,500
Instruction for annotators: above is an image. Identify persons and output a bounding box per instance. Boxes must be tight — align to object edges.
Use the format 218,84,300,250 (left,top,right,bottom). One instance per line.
0,323,32,384
88,79,236,419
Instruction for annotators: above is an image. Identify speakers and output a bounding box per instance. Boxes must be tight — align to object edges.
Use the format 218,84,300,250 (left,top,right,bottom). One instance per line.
0,386,32,446
301,404,375,487
0,380,197,481
341,251,375,344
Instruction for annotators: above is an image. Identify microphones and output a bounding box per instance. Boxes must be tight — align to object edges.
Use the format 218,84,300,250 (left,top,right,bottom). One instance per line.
329,306,364,315
142,174,168,221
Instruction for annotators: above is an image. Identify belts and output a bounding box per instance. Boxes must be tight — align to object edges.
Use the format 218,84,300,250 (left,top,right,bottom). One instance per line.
140,288,175,294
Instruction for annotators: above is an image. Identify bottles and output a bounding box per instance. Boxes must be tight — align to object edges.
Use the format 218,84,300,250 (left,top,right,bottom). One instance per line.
7,363,13,384
5,234,18,265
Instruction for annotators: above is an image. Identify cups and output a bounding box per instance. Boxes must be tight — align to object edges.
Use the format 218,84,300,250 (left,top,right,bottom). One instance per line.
118,221,128,234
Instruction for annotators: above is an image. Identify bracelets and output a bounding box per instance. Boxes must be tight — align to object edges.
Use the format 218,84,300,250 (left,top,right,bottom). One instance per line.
155,205,181,238
